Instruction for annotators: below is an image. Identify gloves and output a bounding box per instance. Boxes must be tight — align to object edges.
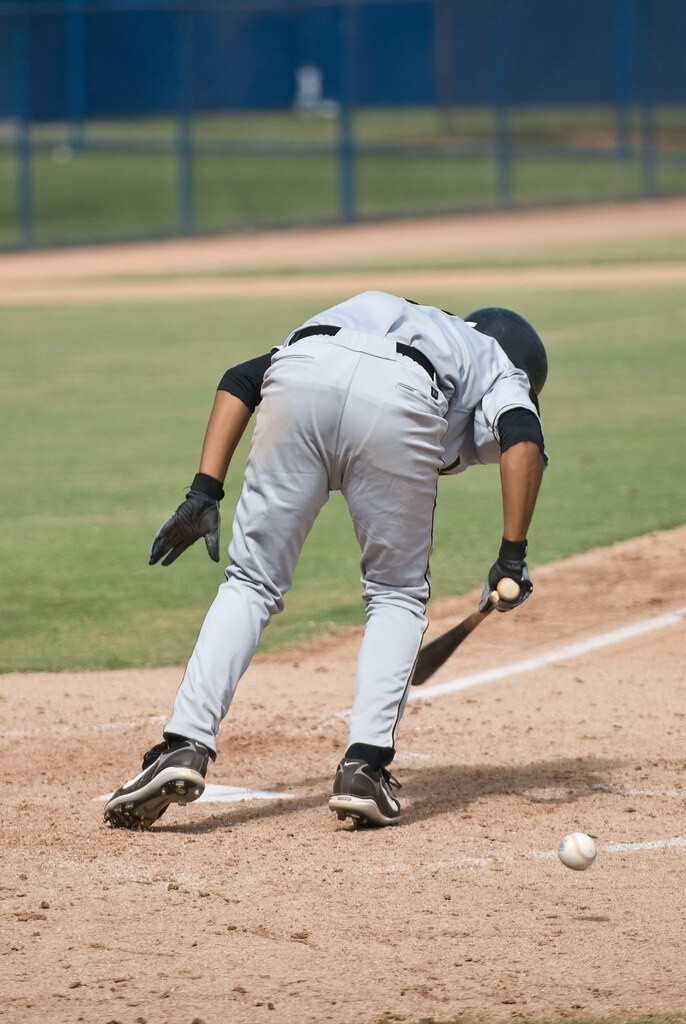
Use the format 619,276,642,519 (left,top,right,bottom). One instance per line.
480,533,533,614
150,472,224,566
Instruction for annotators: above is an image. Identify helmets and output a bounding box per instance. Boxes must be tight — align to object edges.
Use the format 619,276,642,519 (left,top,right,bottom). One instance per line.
464,307,548,417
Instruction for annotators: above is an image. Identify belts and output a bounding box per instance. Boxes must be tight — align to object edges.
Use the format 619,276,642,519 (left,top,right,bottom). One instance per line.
289,325,439,386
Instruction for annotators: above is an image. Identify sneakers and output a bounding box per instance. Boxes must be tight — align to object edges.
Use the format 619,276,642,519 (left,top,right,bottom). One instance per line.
103,735,209,830
329,758,403,825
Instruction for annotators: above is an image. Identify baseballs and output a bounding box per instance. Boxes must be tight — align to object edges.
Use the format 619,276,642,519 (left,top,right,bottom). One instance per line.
558,832,597,871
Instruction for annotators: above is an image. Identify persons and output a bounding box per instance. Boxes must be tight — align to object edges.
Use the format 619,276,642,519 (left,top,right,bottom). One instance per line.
102,290,552,835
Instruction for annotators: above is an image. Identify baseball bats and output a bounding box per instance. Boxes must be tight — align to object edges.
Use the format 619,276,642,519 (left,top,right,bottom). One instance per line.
409,576,523,685
